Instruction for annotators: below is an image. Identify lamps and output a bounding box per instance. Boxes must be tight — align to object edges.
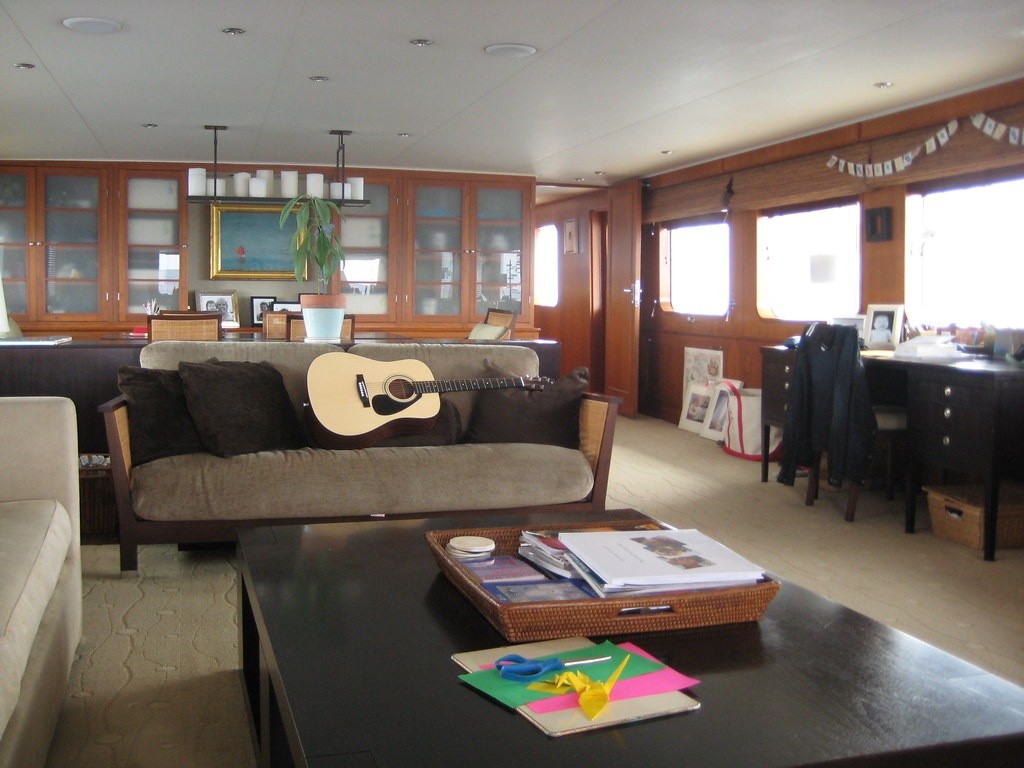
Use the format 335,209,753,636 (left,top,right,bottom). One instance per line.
186,125,372,209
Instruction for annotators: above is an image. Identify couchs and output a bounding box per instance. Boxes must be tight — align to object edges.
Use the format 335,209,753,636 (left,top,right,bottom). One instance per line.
0,395,87,768
96,340,624,579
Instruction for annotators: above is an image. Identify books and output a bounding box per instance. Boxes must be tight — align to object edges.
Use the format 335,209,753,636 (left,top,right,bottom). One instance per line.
456,523,765,605
0,335,73,346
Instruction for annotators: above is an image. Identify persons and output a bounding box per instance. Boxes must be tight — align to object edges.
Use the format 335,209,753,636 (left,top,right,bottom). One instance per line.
206,298,234,321
256,302,269,321
870,315,892,344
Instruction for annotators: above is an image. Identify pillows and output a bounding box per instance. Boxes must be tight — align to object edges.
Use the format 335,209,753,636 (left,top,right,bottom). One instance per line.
463,359,591,451
177,360,307,458
118,365,207,467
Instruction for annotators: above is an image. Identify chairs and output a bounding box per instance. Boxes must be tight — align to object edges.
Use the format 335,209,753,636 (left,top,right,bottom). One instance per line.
463,308,518,341
284,315,356,345
798,321,911,523
147,314,222,342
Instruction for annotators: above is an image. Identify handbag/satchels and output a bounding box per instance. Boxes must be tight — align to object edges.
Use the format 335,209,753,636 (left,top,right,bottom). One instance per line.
703,379,785,461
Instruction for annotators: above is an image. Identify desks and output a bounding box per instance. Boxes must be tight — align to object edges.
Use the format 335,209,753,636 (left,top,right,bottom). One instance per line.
758,344,1024,563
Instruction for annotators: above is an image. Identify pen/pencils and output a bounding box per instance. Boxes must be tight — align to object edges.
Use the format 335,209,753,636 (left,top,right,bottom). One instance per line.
143,298,160,315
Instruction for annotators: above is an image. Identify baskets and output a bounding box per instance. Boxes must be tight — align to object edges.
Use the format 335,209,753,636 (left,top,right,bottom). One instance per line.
424,518,783,641
921,479,1024,549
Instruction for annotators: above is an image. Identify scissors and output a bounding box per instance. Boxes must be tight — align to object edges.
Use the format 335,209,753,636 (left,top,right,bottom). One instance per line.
494,654,612,681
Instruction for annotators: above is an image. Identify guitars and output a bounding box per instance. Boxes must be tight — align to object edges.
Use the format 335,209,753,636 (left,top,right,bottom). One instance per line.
303,352,556,450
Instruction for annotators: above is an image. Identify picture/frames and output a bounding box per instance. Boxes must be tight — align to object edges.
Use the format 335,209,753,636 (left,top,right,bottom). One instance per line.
865,303,905,351
271,302,303,313
207,204,311,282
195,290,241,328
249,295,277,327
562,217,579,253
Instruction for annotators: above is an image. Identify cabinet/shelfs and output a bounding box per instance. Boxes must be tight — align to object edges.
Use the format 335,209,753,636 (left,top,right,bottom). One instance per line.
0,158,541,343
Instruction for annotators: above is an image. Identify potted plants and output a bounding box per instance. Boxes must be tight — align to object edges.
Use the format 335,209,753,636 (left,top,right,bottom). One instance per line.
278,191,348,343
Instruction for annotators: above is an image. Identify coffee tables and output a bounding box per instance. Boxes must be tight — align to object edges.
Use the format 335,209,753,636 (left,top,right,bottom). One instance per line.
235,506,1024,768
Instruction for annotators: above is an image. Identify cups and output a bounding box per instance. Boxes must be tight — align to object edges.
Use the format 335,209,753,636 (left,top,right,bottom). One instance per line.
189,168,364,200
955,330,977,346
920,329,937,336
